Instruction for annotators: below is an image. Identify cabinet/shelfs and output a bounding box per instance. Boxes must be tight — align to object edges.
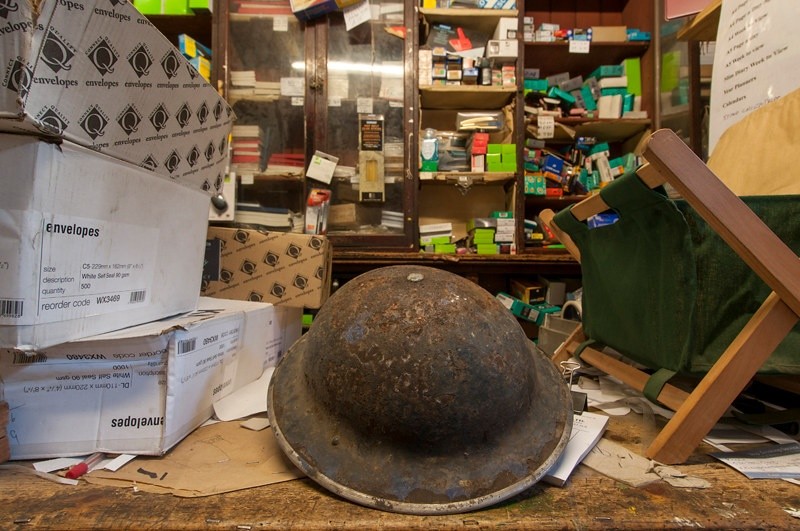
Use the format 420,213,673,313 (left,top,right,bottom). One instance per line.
210,1,660,263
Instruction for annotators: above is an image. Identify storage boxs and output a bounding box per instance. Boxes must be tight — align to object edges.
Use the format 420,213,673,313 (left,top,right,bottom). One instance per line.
0,0,713,460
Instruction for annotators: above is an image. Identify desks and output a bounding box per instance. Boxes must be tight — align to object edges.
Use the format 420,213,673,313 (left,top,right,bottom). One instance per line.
0,374,800,531
